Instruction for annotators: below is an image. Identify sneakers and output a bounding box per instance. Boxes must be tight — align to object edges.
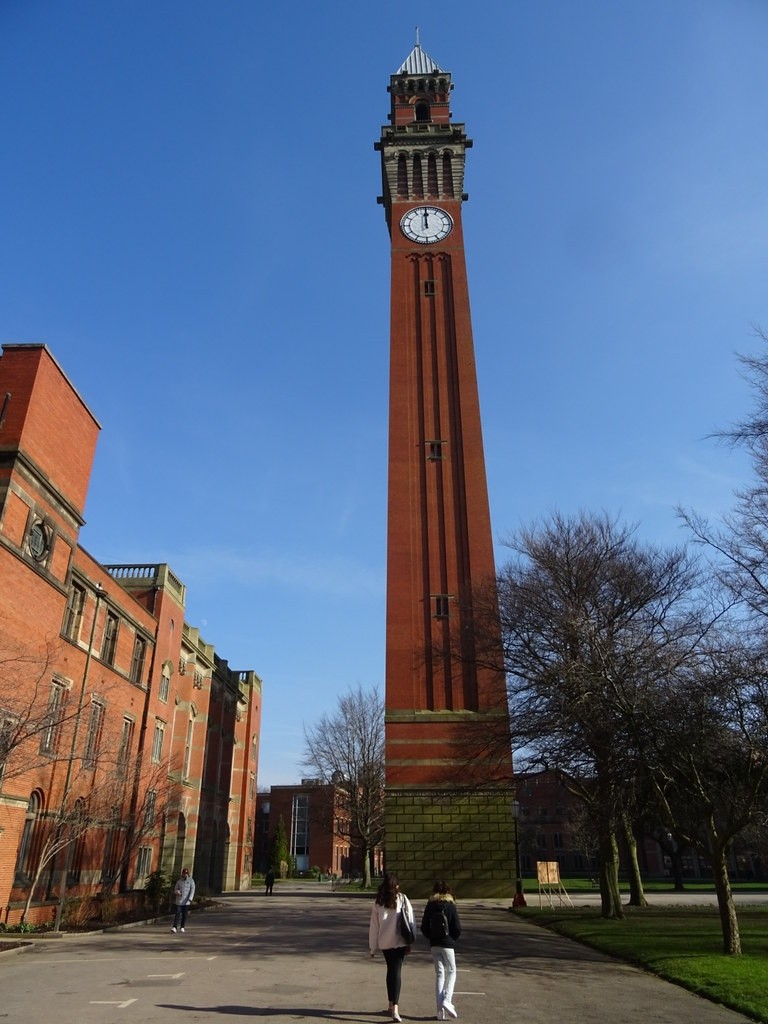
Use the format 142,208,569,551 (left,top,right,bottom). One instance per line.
171,927,176,933
388,1008,394,1017
437,1012,445,1021
442,1000,457,1018
180,928,185,933
393,1014,403,1022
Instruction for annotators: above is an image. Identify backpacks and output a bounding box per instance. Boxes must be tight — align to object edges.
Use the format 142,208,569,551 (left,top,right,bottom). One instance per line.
428,911,449,941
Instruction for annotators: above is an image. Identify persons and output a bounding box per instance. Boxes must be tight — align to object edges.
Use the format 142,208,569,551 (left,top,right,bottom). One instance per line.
369,874,417,1022
327,866,332,879
170,868,196,933
265,871,275,896
346,874,356,885
420,879,461,1020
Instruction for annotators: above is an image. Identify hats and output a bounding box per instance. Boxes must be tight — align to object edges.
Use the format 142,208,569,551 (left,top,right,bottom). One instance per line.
182,868,188,875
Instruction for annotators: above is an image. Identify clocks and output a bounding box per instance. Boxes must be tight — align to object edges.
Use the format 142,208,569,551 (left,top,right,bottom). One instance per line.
400,204,454,245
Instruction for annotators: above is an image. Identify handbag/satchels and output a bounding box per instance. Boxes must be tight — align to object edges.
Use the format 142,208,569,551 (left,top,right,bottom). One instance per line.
400,893,413,944
